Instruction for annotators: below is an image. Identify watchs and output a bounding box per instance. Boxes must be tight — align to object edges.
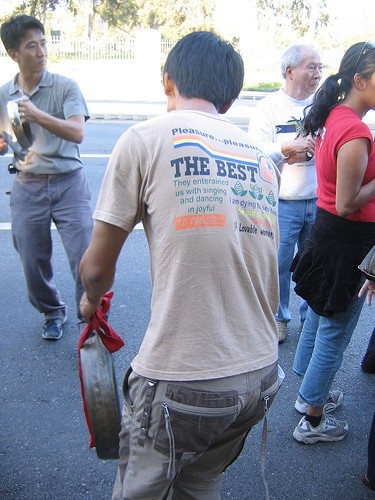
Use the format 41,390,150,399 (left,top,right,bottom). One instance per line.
306,152,313,161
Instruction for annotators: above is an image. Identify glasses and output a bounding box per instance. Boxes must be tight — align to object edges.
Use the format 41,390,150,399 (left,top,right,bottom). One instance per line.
352,38,375,83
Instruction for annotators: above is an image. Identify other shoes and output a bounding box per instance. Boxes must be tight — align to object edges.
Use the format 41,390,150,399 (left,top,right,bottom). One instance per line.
362,466,375,490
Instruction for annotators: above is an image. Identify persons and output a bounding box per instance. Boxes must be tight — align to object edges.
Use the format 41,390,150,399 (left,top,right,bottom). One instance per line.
360,326,375,374
358,245,375,490
80,30,285,500
0,15,93,340
289,42,375,444
248,44,324,342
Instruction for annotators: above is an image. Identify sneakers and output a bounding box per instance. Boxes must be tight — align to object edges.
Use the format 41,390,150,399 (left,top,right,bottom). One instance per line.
294,389,344,414
293,402,349,445
40,313,68,340
275,320,288,343
